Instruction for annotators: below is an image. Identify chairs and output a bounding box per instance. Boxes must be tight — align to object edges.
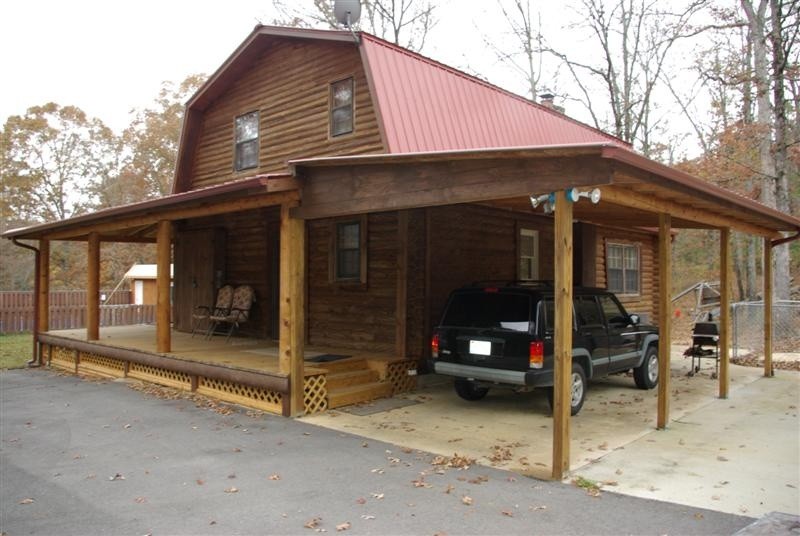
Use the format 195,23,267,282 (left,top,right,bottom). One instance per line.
684,323,720,379
191,285,262,345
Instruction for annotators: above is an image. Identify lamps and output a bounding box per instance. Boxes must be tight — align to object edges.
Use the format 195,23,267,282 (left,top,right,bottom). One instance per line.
530,191,556,213
566,186,601,204
334,0,362,46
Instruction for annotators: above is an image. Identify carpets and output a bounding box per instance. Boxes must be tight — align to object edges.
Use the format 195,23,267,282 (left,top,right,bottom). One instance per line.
304,354,352,362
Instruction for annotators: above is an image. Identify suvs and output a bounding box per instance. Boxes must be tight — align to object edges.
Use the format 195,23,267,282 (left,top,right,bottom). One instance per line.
426,279,660,417
